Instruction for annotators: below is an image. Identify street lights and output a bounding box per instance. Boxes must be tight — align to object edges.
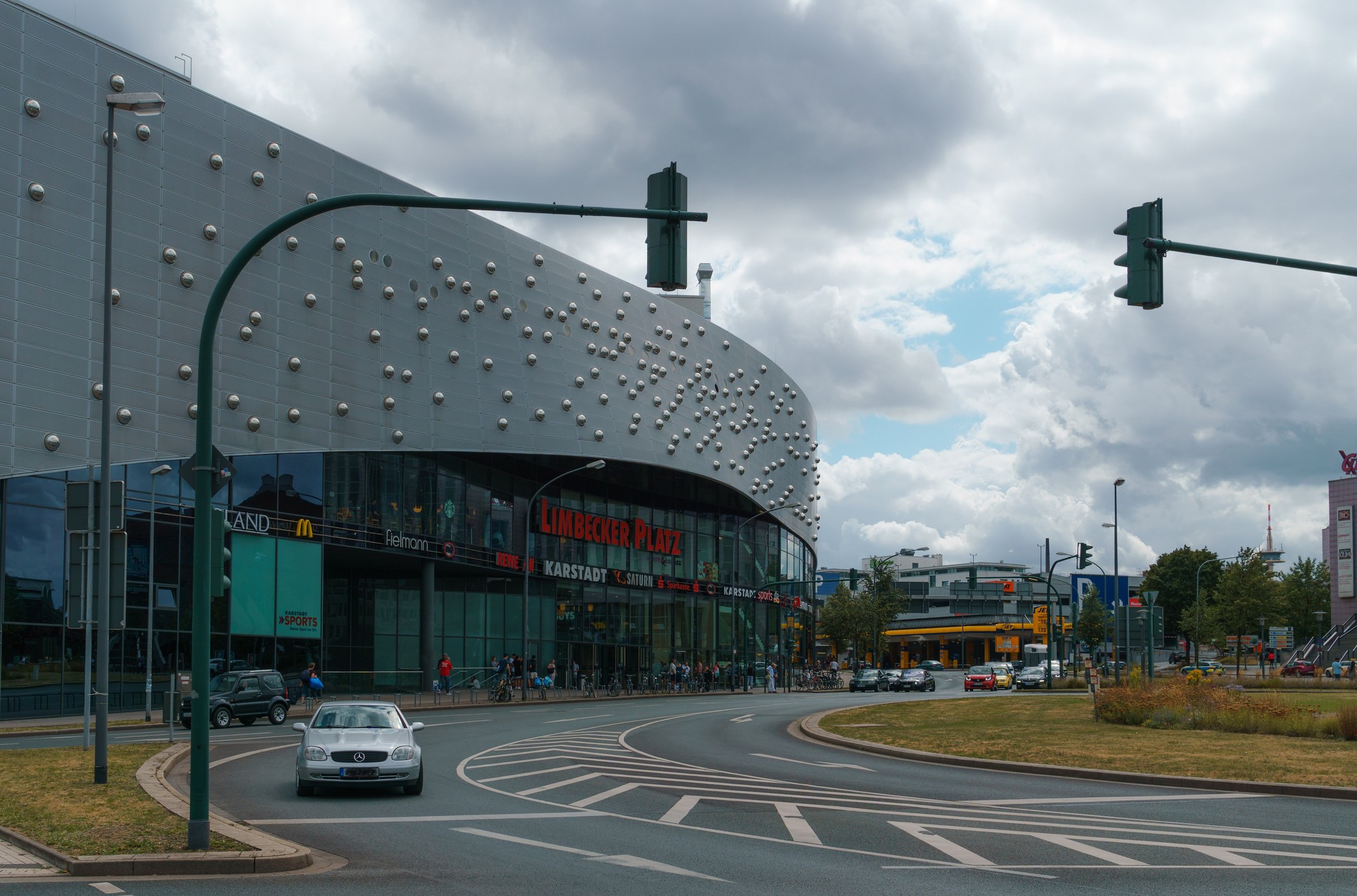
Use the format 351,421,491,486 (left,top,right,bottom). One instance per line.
1056,552,1107,669
90,90,165,785
731,501,801,692
1311,610,1328,668
873,546,931,669
1254,614,1271,679
521,459,608,702
1102,477,1126,688
1196,554,1245,680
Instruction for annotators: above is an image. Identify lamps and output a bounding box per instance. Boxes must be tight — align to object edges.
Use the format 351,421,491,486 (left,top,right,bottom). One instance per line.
959,639,961,641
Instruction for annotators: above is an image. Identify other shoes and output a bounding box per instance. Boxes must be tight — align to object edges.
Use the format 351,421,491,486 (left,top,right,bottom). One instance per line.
512,686,516,689
671,688,676,691
705,690,712,692
446,692,452,695
750,688,753,689
768,691,778,693
549,686,555,689
515,687,521,689
532,686,538,689
529,686,533,690
316,701,322,704
301,702,306,705
436,690,441,694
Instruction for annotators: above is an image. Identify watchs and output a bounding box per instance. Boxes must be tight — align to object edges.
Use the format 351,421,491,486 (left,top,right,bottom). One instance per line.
576,670,577,671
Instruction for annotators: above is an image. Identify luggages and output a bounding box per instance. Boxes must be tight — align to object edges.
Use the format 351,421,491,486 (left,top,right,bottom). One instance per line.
288,686,303,705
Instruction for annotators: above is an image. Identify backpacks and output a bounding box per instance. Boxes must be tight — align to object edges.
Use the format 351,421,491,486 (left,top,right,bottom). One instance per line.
300,669,313,682
496,658,507,673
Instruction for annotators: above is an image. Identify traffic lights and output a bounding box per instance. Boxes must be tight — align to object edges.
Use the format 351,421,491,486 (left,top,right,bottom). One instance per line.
748,636,754,648
1153,614,1163,636
211,506,232,598
1079,543,1093,569
787,638,794,649
1113,199,1168,310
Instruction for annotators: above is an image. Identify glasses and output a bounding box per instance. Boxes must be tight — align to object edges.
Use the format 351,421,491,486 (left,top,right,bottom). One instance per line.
685,661,688,663
817,662,820,663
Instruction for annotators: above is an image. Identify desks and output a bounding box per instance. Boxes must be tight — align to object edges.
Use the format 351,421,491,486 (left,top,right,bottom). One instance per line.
352,509,361,524
407,518,416,533
325,504,334,519
379,513,388,527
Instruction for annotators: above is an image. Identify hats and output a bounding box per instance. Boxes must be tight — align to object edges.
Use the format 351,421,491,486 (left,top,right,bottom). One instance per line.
512,653,517,656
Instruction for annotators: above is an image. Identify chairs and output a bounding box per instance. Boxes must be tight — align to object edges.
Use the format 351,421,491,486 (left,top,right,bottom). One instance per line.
492,538,503,550
336,507,422,534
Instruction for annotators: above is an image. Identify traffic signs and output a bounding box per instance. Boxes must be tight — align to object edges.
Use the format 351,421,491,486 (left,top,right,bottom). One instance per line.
1269,626,1294,649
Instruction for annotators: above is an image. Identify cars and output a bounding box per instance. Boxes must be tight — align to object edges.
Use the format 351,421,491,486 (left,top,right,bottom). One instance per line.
1180,659,1226,677
1097,661,1127,675
206,658,254,675
747,662,769,677
849,668,936,693
1282,661,1317,677
963,661,1018,692
1325,660,1357,678
292,700,427,797
1169,652,1188,664
914,660,944,671
849,660,873,670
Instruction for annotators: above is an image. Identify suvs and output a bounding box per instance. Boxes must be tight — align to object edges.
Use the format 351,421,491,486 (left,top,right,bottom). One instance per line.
179,668,291,730
1009,660,1026,671
1016,659,1068,690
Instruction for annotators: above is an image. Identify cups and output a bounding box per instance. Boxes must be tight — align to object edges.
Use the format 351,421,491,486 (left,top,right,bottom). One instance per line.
574,671,575,673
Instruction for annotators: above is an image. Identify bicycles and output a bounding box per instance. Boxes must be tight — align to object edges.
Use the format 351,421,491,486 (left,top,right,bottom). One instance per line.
795,671,845,690
582,671,710,699
490,676,516,702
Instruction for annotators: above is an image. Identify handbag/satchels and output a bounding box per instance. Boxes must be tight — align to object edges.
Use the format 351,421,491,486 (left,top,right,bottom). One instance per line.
509,668,512,678
1258,661,1259,667
547,664,555,674
310,674,324,690
829,668,837,674
534,676,552,688
765,674,771,680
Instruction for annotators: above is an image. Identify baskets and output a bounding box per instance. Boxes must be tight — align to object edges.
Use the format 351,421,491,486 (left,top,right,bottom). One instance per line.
664,672,671,678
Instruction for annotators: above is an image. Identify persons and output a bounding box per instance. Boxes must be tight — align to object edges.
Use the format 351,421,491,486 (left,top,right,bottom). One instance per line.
547,659,558,689
437,652,453,695
299,663,323,705
829,659,839,681
238,679,251,690
466,674,480,689
766,663,778,693
747,664,753,690
491,653,538,695
825,655,838,672
803,659,823,690
726,663,732,689
670,659,691,690
1332,657,1345,682
574,661,579,689
694,659,713,692
1259,651,1274,669
1343,661,1357,681
714,662,719,690
652,659,669,690
852,657,861,676
